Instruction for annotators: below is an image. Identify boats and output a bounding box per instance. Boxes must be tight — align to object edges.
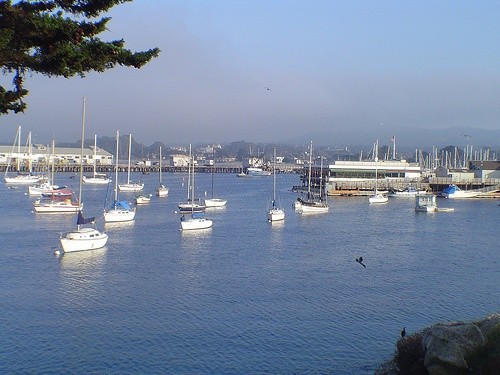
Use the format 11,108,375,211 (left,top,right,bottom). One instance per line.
323,135,422,195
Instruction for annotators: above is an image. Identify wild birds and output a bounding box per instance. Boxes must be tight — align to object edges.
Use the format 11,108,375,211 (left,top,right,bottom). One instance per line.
400,327,407,338
356,256,367,268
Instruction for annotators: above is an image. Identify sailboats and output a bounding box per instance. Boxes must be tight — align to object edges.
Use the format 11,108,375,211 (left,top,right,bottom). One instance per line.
59,97,109,254
367,161,455,213
180,155,214,230
133,142,153,205
83,134,113,185
266,145,286,222
28,138,84,214
102,129,137,223
3,123,84,202
177,157,206,213
154,146,169,197
292,141,331,214
236,159,272,179
203,147,227,207
117,133,145,192
